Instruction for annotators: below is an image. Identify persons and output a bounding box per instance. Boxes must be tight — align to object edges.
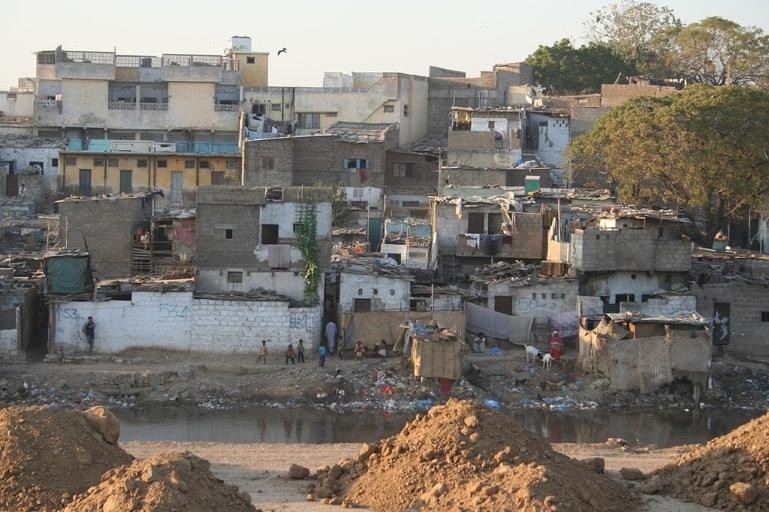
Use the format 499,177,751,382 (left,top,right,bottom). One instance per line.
18,183,26,197
284,342,296,365
80,316,96,353
354,340,365,359
297,338,306,365
255,340,269,366
548,329,565,360
318,343,327,368
141,231,150,241
325,319,338,355
471,331,488,353
374,338,388,357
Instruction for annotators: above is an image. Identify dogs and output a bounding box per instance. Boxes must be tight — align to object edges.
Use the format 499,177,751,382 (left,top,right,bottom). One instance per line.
539,353,556,369
523,343,542,364
